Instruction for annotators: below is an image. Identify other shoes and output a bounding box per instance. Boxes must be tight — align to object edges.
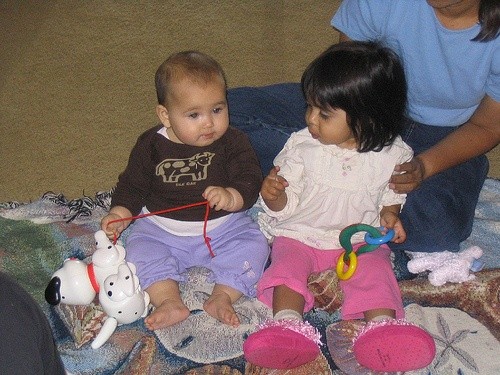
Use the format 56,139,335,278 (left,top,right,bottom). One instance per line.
243,317,324,368
351,319,436,370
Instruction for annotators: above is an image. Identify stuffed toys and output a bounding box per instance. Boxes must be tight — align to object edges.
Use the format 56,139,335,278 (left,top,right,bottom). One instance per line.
404,244,483,286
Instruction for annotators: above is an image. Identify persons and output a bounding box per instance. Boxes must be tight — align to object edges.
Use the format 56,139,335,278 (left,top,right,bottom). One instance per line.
0,271,60,375
242,38,435,371
101,49,269,331
329,0,500,251
